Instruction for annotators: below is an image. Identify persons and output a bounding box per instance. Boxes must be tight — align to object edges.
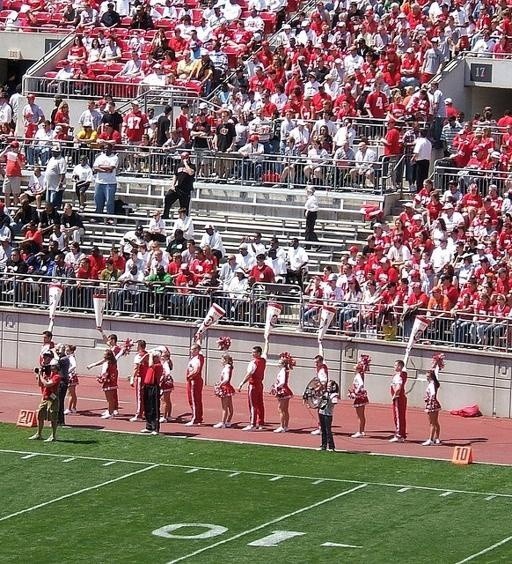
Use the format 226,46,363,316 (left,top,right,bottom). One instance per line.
87,326,174,423
350,365,369,438
140,351,163,435
273,358,293,432
238,346,266,430
0,3,512,352
185,344,204,427
213,348,235,427
389,360,407,442
311,354,328,435
423,363,441,446
308,380,339,452
28,331,79,442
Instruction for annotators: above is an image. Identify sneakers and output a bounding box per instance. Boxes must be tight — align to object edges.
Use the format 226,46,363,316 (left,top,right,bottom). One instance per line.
184,422,201,427
129,416,146,422
63,408,76,414
140,429,159,435
28,434,40,439
43,436,55,443
388,437,405,442
316,447,334,452
351,432,365,438
273,427,288,432
159,417,172,423
311,430,322,435
100,410,118,418
241,425,263,431
213,422,231,429
422,439,440,446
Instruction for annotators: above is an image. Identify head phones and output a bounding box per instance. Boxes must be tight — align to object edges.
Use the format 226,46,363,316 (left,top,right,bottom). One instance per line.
333,381,336,391
